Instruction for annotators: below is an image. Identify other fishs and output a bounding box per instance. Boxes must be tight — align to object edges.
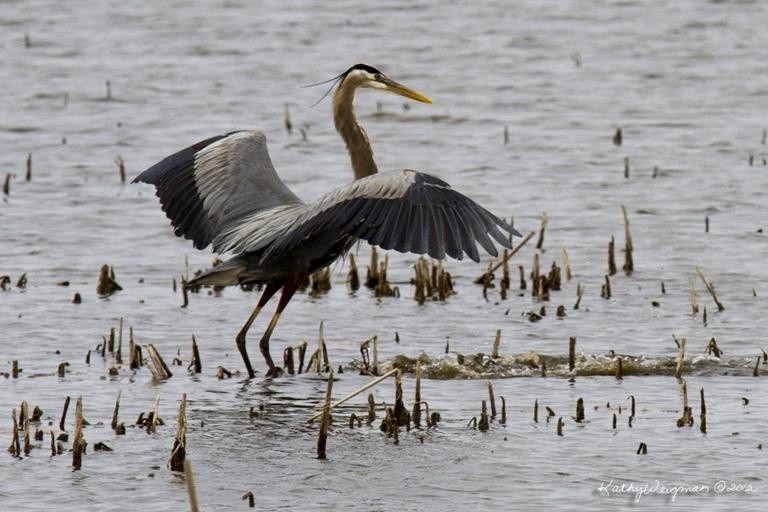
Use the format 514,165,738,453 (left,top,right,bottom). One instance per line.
130,63,523,377
